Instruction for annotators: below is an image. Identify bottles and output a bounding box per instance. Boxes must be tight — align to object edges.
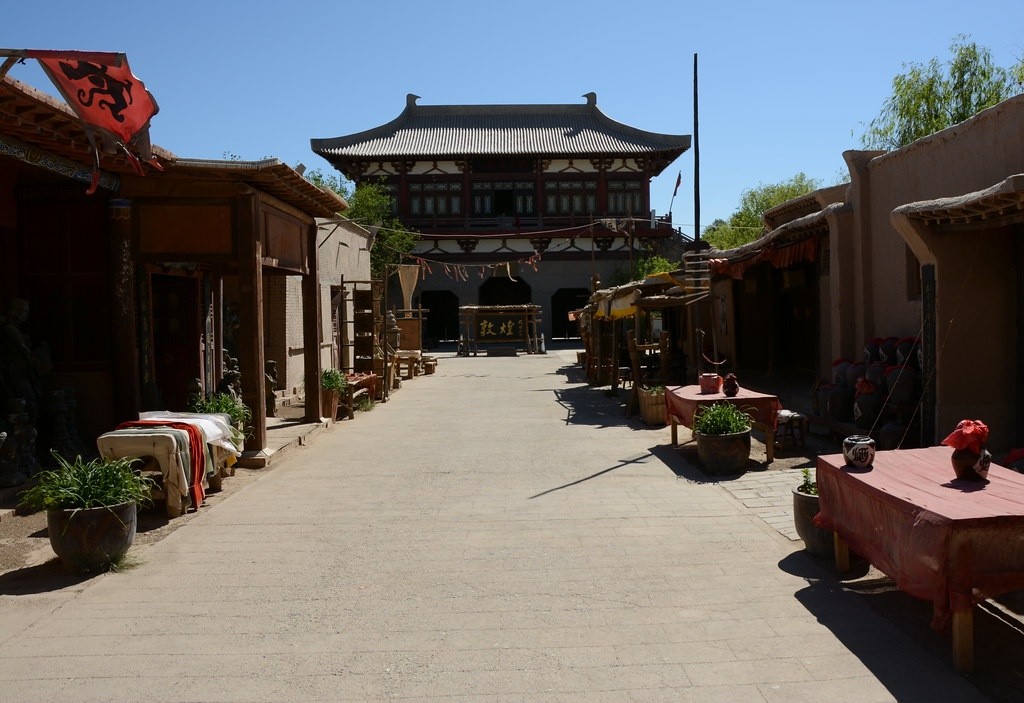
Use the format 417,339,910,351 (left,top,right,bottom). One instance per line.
951,442,991,482
723,373,739,397
699,372,720,394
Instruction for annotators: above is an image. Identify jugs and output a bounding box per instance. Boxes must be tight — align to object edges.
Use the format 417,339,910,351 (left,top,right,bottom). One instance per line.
843,434,876,470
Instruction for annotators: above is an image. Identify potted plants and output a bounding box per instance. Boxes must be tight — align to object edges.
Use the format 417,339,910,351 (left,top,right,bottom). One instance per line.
187,390,255,478
304,368,347,424
791,468,827,557
691,398,755,477
14,448,161,573
637,382,671,427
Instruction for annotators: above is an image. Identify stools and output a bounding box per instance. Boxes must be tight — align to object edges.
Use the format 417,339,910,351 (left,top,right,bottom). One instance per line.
423,358,438,366
424,362,435,374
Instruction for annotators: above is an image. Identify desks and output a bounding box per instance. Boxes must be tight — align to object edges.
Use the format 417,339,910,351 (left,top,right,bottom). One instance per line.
97,411,230,517
817,445,1024,672
664,384,779,465
